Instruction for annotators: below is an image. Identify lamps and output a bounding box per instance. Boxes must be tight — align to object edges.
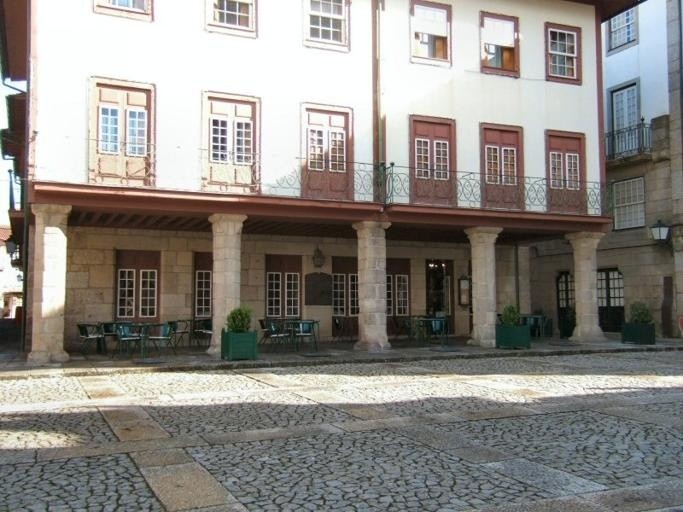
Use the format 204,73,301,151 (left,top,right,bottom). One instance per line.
458,275,471,310
649,219,672,246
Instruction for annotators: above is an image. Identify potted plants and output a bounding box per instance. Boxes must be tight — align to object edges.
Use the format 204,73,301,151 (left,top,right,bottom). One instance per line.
495,304,531,350
220,308,257,362
621,302,656,345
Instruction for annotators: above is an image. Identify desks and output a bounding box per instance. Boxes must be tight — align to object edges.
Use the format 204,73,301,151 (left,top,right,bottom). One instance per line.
520,314,545,338
415,317,446,347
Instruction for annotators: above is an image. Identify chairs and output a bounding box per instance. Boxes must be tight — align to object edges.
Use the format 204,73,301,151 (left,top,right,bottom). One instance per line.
256,317,320,355
76,317,212,360
